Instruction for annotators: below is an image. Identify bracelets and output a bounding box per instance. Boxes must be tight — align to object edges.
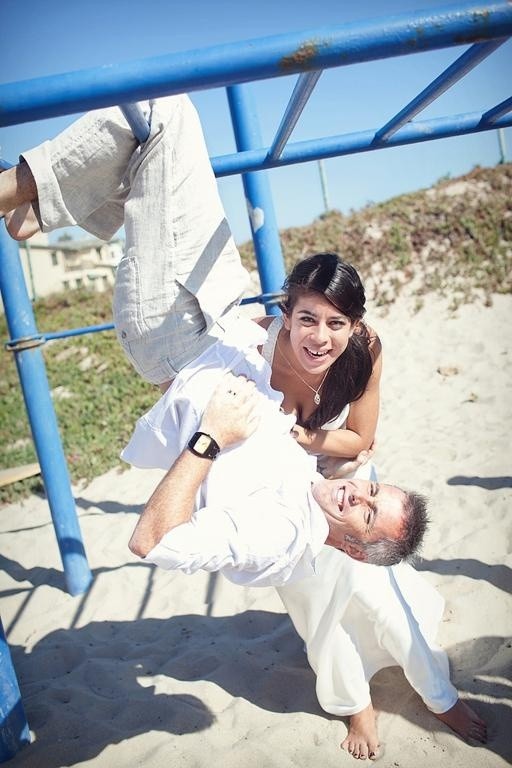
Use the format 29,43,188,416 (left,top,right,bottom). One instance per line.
183,428,225,467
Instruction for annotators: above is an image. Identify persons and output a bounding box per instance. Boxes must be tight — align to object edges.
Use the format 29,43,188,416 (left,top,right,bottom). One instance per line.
0,91,430,591
246,251,490,760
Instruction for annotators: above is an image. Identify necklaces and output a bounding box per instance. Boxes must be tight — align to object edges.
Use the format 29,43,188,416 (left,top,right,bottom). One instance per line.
273,341,336,406
